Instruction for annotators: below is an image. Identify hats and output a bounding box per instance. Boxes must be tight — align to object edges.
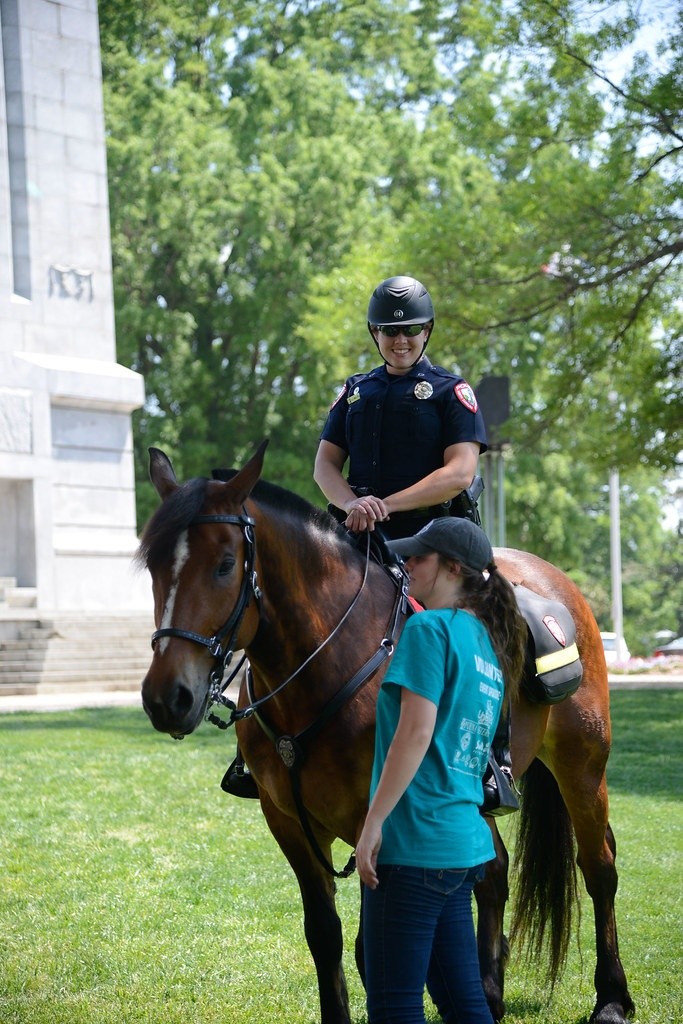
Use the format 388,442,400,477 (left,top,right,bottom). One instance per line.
384,516,494,573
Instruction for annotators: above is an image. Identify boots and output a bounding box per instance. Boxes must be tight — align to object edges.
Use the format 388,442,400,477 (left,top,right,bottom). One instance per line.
483,688,522,808
228,768,258,794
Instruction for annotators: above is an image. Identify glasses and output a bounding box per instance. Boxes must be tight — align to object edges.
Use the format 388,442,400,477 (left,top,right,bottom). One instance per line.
377,324,427,337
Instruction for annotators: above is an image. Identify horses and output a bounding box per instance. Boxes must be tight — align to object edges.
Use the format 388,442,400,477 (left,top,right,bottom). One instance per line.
142,439,636,1024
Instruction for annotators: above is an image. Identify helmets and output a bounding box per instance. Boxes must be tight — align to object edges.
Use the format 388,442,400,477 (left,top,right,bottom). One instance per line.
368,276,434,325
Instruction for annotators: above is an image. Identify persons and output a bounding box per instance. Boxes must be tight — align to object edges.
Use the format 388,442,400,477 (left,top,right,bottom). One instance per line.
356,517,528,1024
226,275,520,811
